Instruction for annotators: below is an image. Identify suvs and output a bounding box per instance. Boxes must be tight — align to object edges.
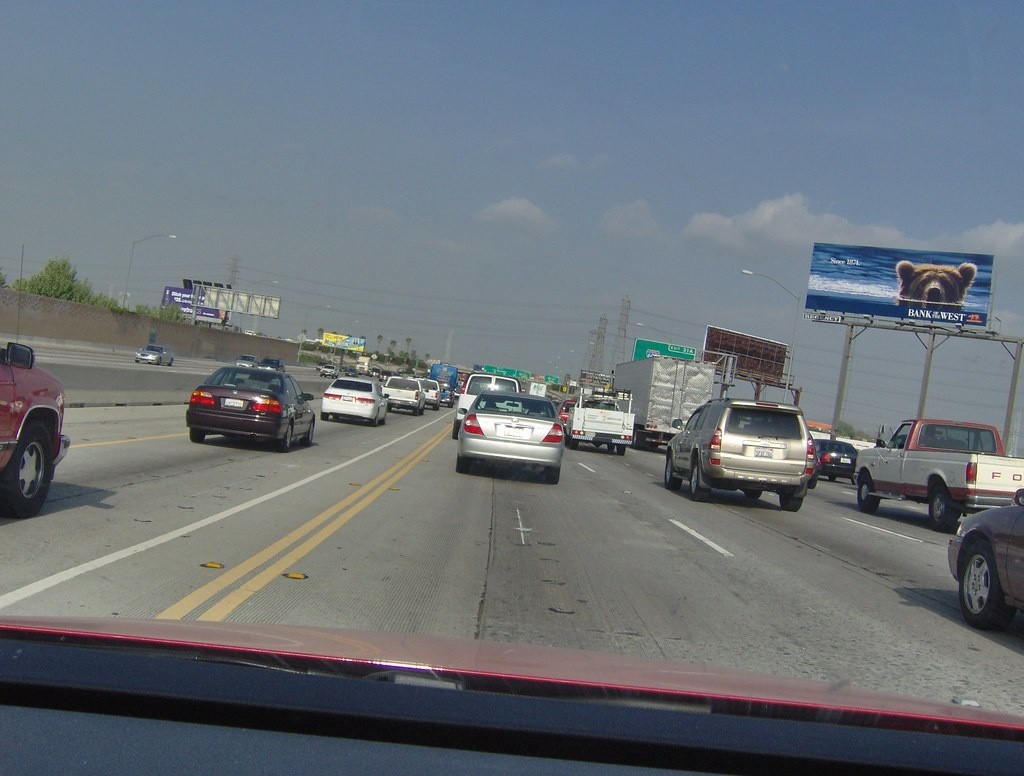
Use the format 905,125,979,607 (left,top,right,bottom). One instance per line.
0,341,71,521
414,378,440,411
557,400,576,432
665,396,816,513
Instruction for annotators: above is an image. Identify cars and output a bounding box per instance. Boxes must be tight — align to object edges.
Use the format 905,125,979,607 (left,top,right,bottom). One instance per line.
316,356,404,382
811,438,859,486
947,488,1024,635
135,345,174,365
186,365,316,453
235,354,260,368
256,358,286,373
320,376,390,427
437,382,456,408
455,389,565,486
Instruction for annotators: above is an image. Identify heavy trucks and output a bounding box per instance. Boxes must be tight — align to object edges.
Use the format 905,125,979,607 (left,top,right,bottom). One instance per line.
611,356,717,452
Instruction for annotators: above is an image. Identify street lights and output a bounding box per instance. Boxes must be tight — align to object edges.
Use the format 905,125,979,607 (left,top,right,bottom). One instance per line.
245,278,279,292
296,304,331,362
740,268,801,404
636,323,672,344
122,234,177,311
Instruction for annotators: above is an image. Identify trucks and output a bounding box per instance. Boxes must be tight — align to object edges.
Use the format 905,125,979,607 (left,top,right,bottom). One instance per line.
430,363,459,391
526,381,546,398
564,391,635,455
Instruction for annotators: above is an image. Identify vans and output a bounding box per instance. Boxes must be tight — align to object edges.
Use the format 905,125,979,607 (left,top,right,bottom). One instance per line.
451,372,525,439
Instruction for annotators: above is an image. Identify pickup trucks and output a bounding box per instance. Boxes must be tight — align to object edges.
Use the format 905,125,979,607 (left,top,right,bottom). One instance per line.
379,375,426,417
853,417,1024,533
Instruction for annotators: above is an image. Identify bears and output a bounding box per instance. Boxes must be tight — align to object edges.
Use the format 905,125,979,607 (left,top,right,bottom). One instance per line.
895,260,978,304
